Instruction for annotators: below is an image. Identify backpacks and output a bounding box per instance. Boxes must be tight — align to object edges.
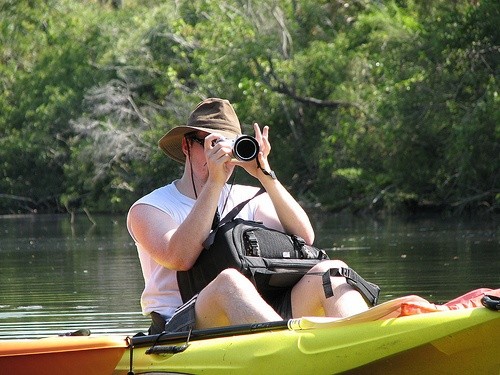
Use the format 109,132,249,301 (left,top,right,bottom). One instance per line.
176,218,380,307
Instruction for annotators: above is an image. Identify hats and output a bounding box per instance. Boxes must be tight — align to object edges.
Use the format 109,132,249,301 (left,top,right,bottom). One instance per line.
158,98,242,164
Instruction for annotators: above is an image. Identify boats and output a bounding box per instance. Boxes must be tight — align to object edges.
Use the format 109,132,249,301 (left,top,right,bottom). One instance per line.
0,295,500,375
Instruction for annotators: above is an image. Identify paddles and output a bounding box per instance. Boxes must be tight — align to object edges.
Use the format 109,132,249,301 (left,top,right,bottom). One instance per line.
0,294,430,358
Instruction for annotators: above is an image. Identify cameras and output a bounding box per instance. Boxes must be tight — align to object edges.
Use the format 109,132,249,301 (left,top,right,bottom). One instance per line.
216,134,260,161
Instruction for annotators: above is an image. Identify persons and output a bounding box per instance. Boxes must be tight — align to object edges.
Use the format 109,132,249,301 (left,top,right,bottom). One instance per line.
125,98,377,335
